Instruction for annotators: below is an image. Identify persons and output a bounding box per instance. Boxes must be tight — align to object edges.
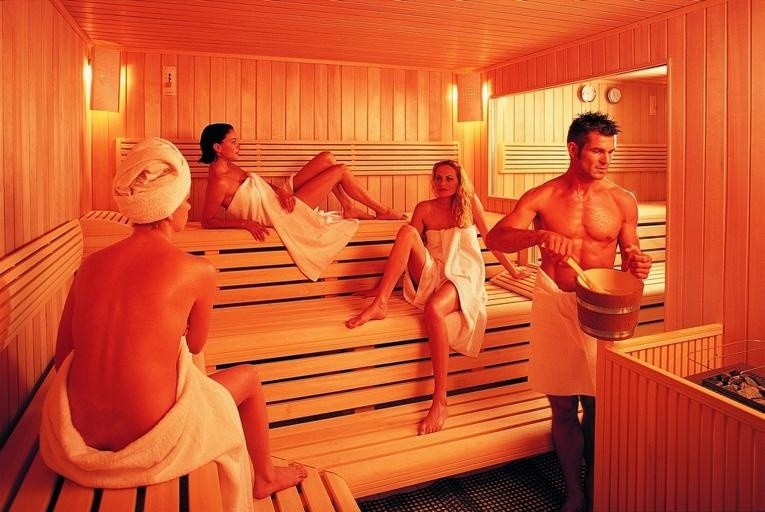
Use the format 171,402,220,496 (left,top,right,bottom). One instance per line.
484,109,652,511
53,137,307,500
345,159,533,437
197,122,408,243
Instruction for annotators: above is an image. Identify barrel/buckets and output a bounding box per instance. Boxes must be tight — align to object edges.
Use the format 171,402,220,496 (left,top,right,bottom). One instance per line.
574,263,646,340
